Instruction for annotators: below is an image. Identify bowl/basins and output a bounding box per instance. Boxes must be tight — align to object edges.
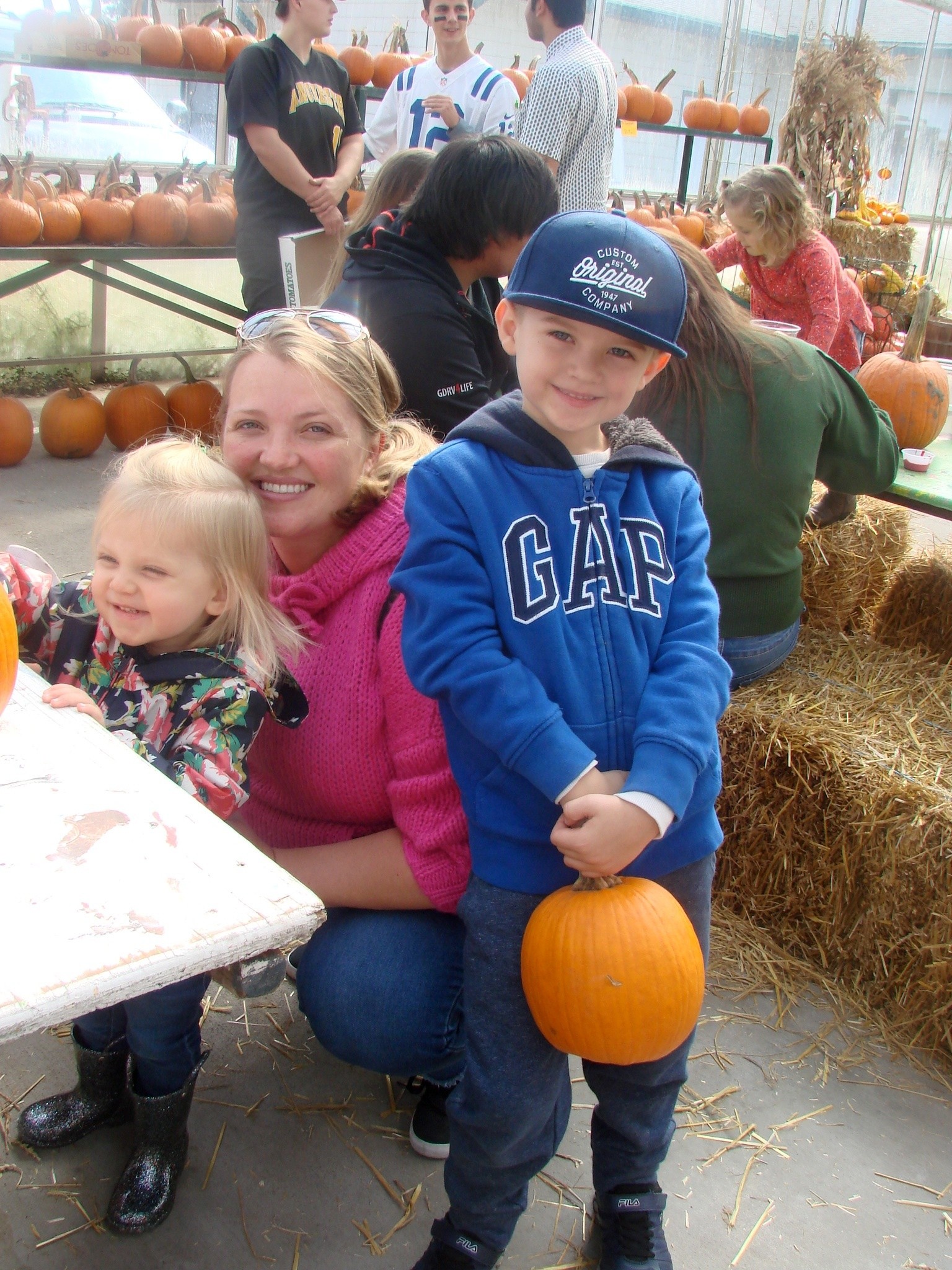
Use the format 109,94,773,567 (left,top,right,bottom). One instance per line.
901,448,935,472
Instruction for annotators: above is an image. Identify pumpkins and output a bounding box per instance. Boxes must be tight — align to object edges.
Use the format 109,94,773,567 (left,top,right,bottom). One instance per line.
0,3,950,467
520,871,705,1066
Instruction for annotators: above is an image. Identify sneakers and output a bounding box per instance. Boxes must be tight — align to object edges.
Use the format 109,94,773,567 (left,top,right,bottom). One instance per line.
412,1211,506,1270
396,1078,461,1159
594,1181,674,1270
285,941,308,980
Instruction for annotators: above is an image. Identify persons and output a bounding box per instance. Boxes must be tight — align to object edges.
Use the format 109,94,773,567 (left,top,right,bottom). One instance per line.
362,1,521,171
203,307,473,1160
696,164,878,530
378,211,741,1270
1,431,325,1236
514,0,620,215
311,125,900,693
225,0,366,321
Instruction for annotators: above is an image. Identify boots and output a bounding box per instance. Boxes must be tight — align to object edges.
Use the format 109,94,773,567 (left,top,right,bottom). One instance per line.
105,1048,211,1235
18,1022,140,1150
804,488,856,530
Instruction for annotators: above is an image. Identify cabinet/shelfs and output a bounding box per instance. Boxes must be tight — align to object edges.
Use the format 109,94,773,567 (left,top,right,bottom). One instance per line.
0,45,772,336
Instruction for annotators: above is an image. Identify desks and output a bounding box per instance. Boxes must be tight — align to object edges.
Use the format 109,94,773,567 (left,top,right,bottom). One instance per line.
868,437,952,521
0,659,327,1050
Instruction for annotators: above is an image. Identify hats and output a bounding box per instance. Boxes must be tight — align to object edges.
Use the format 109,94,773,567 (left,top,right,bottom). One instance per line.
505,208,688,359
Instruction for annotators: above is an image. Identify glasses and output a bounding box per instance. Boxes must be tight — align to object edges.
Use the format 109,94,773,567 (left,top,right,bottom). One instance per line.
236,307,384,395
